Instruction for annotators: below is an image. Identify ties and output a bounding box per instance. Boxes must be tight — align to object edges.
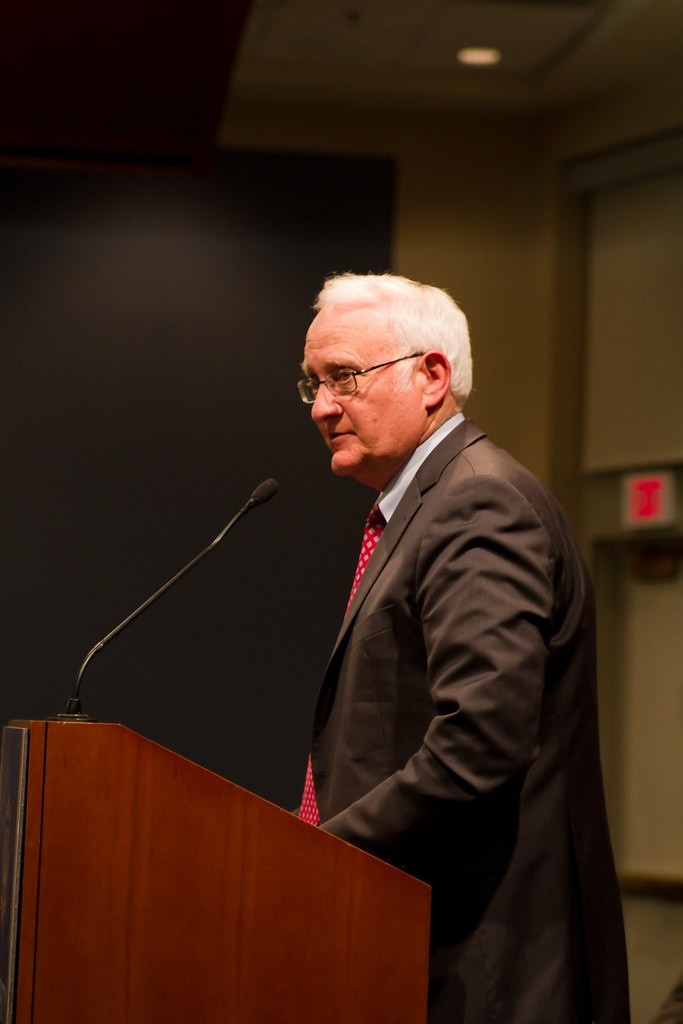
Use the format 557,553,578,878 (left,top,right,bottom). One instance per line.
298,500,388,828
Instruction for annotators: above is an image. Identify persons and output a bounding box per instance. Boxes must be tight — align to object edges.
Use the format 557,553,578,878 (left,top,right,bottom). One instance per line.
300,274,632,1024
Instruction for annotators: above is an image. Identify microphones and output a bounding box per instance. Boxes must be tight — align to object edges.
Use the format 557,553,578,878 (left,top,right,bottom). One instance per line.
51,477,278,723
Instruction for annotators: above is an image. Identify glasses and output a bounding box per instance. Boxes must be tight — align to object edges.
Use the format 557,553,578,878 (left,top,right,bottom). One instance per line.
296,352,428,407
